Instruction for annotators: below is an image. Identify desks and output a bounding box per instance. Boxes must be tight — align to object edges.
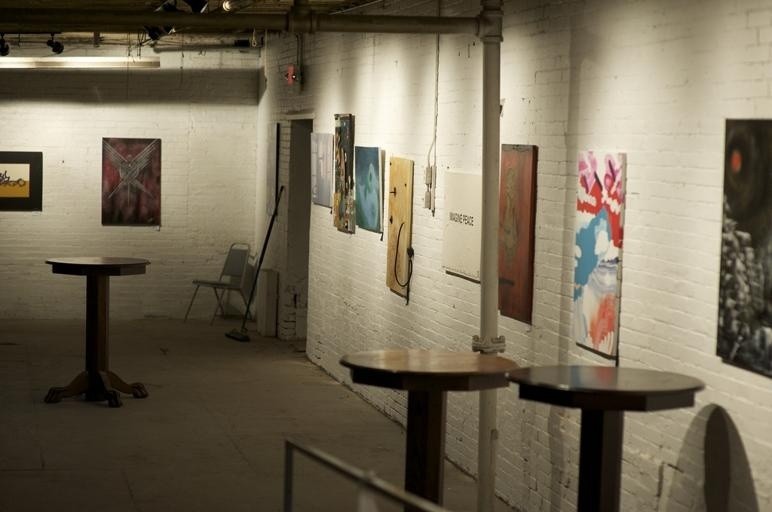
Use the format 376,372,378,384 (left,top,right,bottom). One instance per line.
44,256,153,407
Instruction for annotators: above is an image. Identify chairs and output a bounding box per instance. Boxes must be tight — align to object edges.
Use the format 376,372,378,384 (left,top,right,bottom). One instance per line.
183,242,253,327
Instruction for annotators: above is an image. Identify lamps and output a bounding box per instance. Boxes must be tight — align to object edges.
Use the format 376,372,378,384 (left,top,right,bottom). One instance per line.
183,1,208,14
45,31,64,55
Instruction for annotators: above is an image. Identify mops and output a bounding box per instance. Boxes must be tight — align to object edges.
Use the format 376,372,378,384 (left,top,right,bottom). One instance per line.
226,184,287,343
